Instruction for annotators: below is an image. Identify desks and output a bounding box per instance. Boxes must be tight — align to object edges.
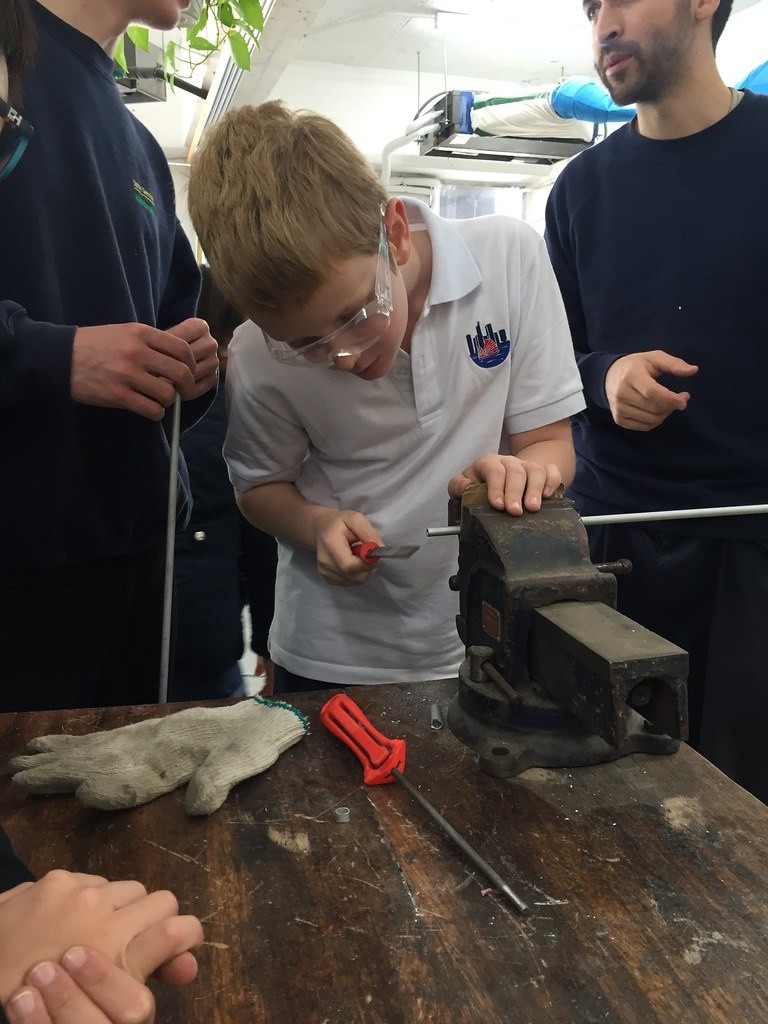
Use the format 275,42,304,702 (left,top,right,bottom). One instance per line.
0,678,768,1024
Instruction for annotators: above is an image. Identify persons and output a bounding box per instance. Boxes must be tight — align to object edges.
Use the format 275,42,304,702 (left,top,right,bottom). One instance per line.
542,0,768,799
189,99,587,696
0,0,276,714
0,828,204,1024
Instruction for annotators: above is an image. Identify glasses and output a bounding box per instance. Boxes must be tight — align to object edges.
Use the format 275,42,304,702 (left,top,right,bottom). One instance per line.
0,97,34,179
260,203,395,368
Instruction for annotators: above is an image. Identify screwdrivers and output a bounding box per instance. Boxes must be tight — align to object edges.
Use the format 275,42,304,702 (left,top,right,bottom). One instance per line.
317,689,533,917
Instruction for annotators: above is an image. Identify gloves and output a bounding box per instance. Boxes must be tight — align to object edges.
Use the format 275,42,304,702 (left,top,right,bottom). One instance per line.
10,694,311,816
